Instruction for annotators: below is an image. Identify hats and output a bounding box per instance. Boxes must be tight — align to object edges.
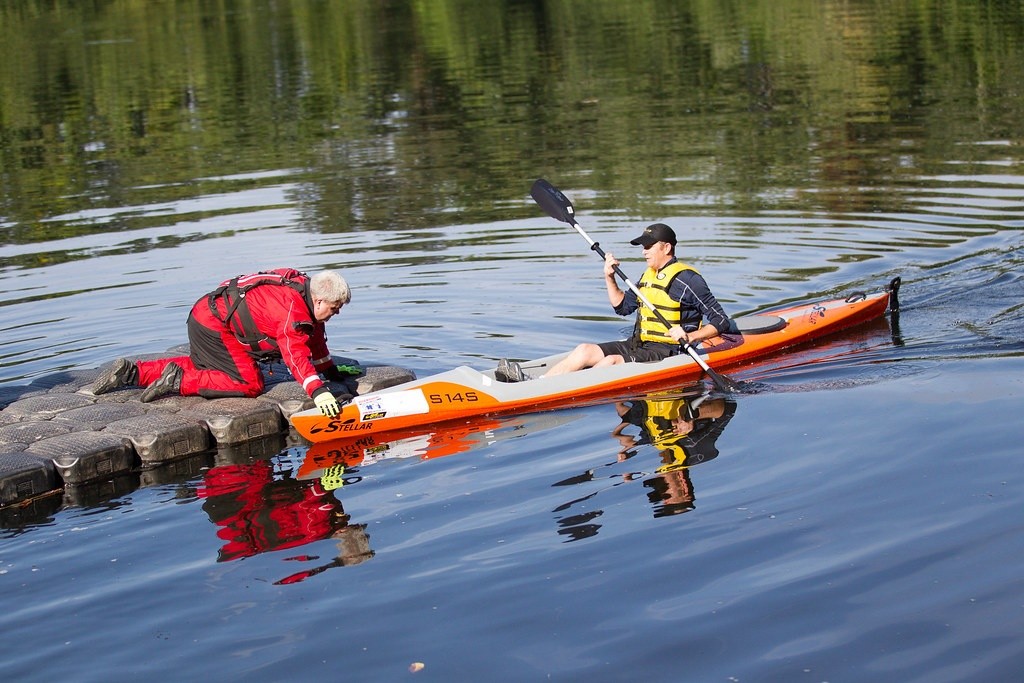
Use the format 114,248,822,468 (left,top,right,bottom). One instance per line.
630,224,677,247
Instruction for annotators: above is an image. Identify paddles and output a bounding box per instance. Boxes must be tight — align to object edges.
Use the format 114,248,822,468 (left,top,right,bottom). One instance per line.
526,174,755,395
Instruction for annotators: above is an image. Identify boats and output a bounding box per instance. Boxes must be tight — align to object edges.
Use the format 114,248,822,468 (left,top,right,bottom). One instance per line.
287,274,903,444
295,313,904,483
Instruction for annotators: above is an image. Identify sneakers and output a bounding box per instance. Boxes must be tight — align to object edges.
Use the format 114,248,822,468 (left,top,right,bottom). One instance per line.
140,361,183,403
93,358,137,395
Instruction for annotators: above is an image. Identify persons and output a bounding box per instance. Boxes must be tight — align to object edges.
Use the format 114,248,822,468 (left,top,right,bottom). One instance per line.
495,223,730,387
609,384,738,516
92,266,352,418
176,458,376,587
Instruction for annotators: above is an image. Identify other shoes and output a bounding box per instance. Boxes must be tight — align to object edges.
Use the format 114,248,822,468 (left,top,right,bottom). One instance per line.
509,362,532,382
497,358,510,378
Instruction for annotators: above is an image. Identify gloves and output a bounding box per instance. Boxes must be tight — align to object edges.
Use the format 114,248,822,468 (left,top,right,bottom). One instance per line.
313,387,341,417
